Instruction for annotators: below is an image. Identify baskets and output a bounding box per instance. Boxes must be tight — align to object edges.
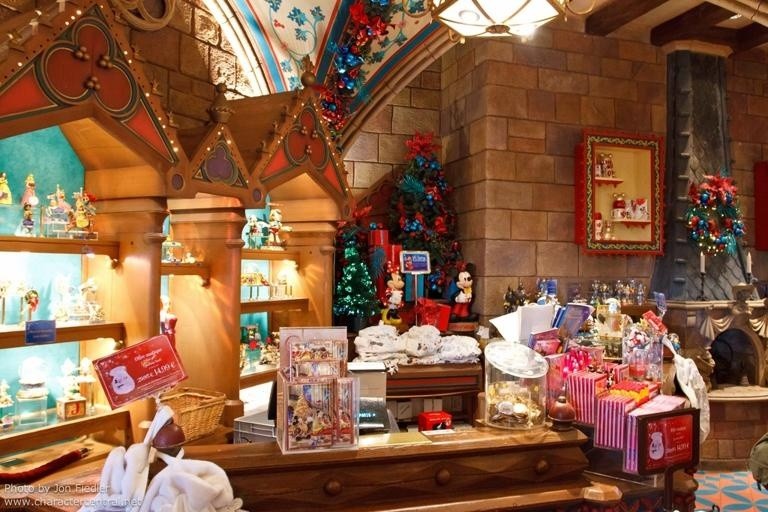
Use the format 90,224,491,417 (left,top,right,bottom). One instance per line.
159,387,228,440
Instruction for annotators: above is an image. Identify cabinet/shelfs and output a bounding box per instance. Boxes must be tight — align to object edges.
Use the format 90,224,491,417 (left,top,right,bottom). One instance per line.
574,129,664,255
0,238,125,505
238,248,310,391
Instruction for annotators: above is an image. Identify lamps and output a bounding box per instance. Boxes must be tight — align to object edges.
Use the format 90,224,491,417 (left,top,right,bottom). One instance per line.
428,0,560,46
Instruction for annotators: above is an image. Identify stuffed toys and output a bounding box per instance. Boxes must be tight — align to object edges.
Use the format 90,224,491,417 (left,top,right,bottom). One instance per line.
0,171,108,434
